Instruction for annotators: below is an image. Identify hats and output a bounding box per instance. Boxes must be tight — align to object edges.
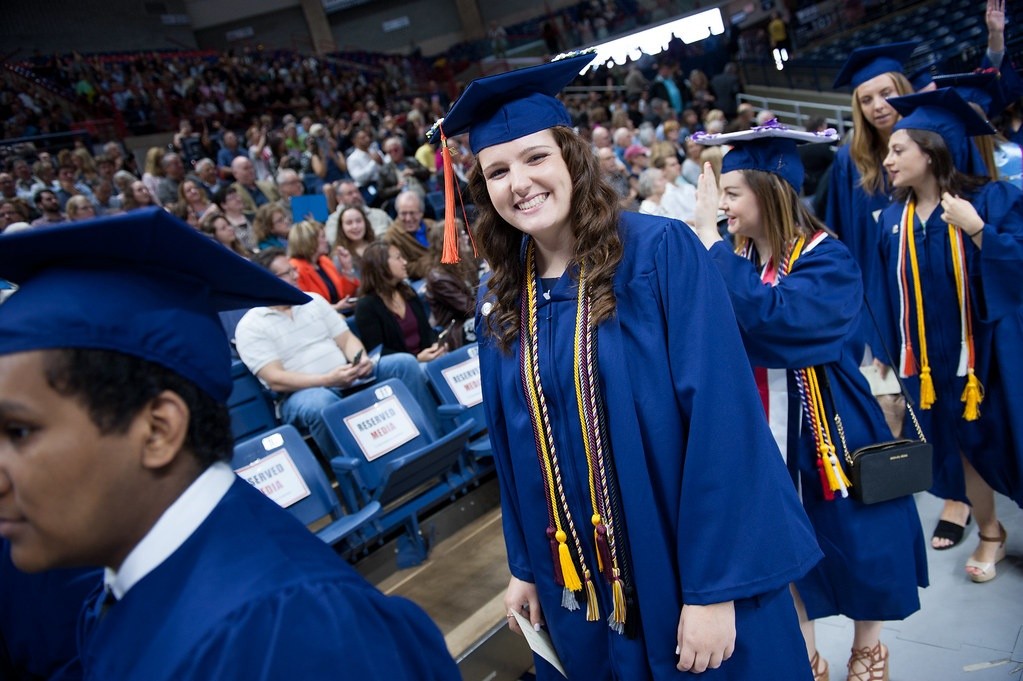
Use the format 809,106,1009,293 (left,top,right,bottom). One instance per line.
690,127,840,197
624,144,649,160
427,50,597,265
886,56,1015,170
832,39,916,90
2,204,315,399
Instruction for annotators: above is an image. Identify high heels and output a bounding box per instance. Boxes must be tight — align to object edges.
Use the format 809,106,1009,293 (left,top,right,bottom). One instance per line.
811,649,833,681
845,639,891,681
965,519,1007,583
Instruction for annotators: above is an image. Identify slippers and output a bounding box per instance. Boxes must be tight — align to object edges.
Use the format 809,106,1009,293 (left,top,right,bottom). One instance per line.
931,505,971,550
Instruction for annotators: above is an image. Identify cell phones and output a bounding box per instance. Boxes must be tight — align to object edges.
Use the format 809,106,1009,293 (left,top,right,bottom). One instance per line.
352,349,362,367
438,328,449,349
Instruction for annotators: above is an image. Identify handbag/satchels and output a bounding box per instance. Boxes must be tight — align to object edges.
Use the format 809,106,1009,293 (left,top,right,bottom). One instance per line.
844,436,933,506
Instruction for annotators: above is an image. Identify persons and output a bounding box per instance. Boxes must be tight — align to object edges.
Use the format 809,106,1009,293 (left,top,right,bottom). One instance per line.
807,0,1023,583
0,205,464,681
428,50,828,681
0,0,1023,463
682,126,930,681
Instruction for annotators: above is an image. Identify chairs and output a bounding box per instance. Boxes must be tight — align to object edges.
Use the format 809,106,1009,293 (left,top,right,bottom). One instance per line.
289,194,331,226
319,377,477,568
791,0,1023,70
424,337,511,457
223,425,381,559
214,306,280,448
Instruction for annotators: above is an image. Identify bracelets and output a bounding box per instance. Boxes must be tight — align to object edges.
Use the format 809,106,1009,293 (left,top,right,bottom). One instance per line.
970,227,983,238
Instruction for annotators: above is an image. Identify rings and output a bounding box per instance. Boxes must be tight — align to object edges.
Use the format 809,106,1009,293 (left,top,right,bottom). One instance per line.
506,614,513,619
